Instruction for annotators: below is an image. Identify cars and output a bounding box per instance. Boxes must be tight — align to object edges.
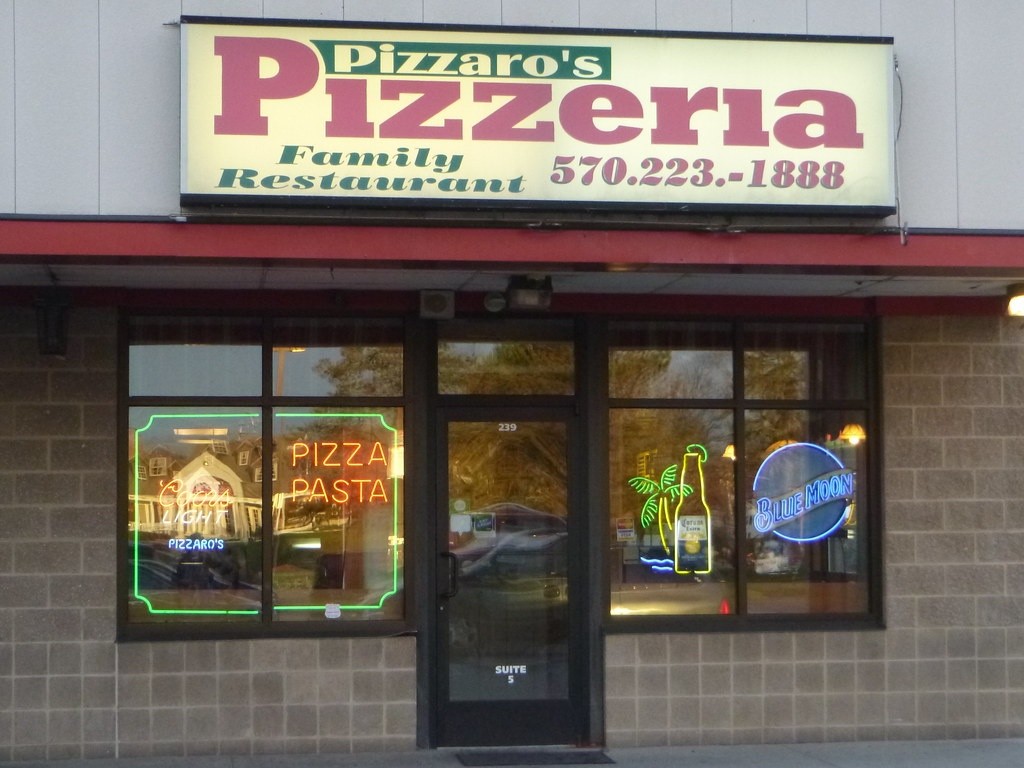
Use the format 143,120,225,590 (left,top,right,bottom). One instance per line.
352,546,496,618
460,529,569,616
274,493,358,570
754,547,790,577
